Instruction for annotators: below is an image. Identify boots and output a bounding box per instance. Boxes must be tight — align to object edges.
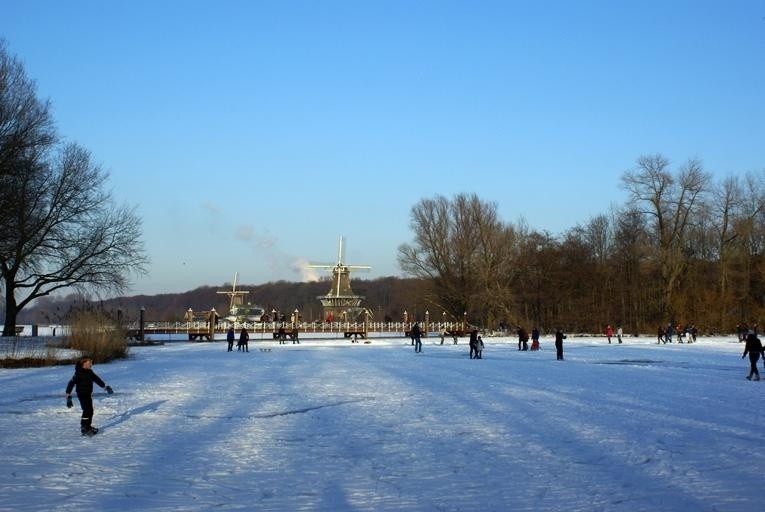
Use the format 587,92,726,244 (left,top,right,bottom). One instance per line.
81,419,99,435
280,340,299,344
745,373,760,381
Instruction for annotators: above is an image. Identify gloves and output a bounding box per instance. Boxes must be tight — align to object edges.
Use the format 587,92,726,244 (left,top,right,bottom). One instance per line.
66,396,74,409
741,352,746,359
105,386,114,396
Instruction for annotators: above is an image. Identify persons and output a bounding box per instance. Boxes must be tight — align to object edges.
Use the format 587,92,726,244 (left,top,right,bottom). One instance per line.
741,330,765,381
439,325,449,345
225,327,234,352
657,321,697,345
291,327,300,344
411,322,424,352
473,336,485,360
239,328,249,352
735,321,760,342
531,327,540,343
65,357,113,436
522,328,529,351
555,328,568,360
469,329,480,359
516,326,527,351
606,323,613,344
616,325,623,343
278,325,286,345
453,331,459,345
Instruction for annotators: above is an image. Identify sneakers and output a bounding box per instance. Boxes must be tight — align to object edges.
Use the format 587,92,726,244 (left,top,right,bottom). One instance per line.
656,339,696,344
470,355,483,359
227,349,248,352
412,344,424,353
518,347,525,351
557,358,564,361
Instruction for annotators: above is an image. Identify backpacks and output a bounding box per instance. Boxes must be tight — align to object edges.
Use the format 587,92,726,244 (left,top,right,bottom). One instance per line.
532,340,539,350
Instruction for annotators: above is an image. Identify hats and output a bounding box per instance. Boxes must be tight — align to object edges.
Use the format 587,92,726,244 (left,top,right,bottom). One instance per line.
747,329,756,335
